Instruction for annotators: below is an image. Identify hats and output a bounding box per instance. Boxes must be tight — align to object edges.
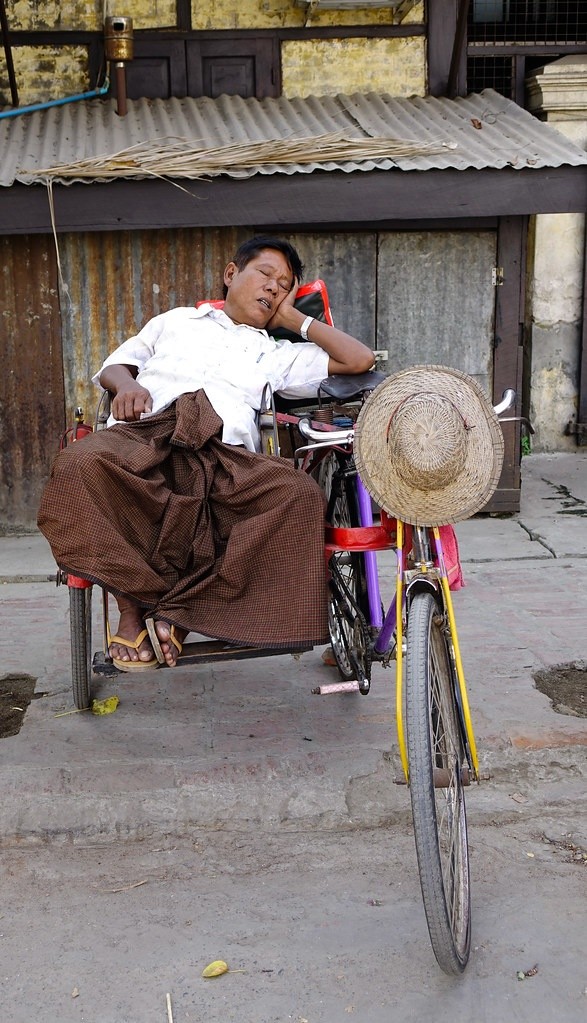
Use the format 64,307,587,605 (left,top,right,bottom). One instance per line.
351,364,506,529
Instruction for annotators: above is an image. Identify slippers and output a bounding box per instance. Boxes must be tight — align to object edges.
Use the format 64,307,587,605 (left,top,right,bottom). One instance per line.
146,617,183,668
109,628,158,674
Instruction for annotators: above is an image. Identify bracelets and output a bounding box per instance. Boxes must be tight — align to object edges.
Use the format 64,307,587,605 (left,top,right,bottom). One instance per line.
300,316,315,341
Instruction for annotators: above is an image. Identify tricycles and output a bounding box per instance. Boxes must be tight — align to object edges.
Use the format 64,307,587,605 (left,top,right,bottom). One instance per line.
42,370,535,975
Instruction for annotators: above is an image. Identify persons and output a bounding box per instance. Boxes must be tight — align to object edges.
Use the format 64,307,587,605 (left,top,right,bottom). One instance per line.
37,234,375,672
